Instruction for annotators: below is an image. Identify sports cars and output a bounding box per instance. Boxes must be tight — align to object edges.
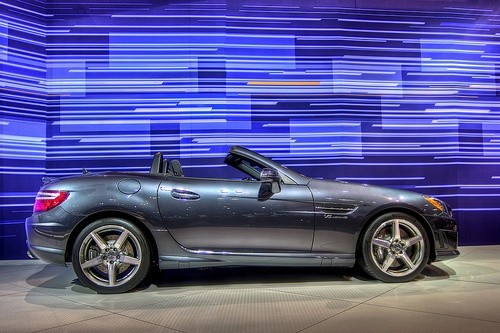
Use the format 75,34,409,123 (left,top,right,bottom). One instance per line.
24,145,460,294
